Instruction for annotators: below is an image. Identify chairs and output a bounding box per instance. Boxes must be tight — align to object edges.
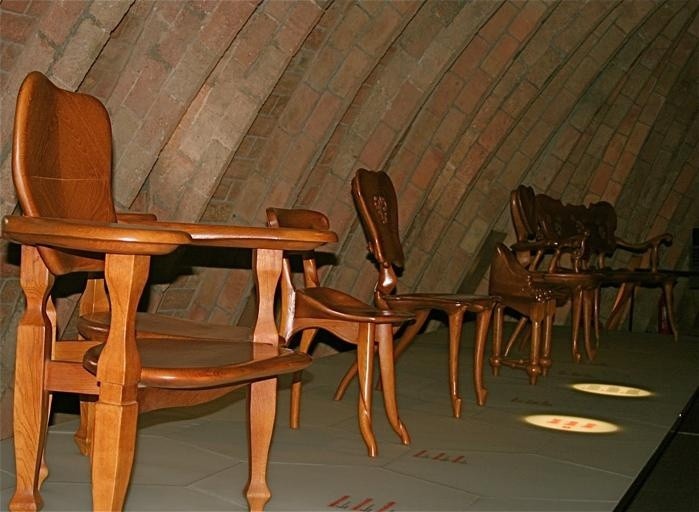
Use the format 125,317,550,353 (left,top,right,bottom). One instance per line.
267,206,408,457
0,60,338,512
334,169,503,418
490,184,683,384
